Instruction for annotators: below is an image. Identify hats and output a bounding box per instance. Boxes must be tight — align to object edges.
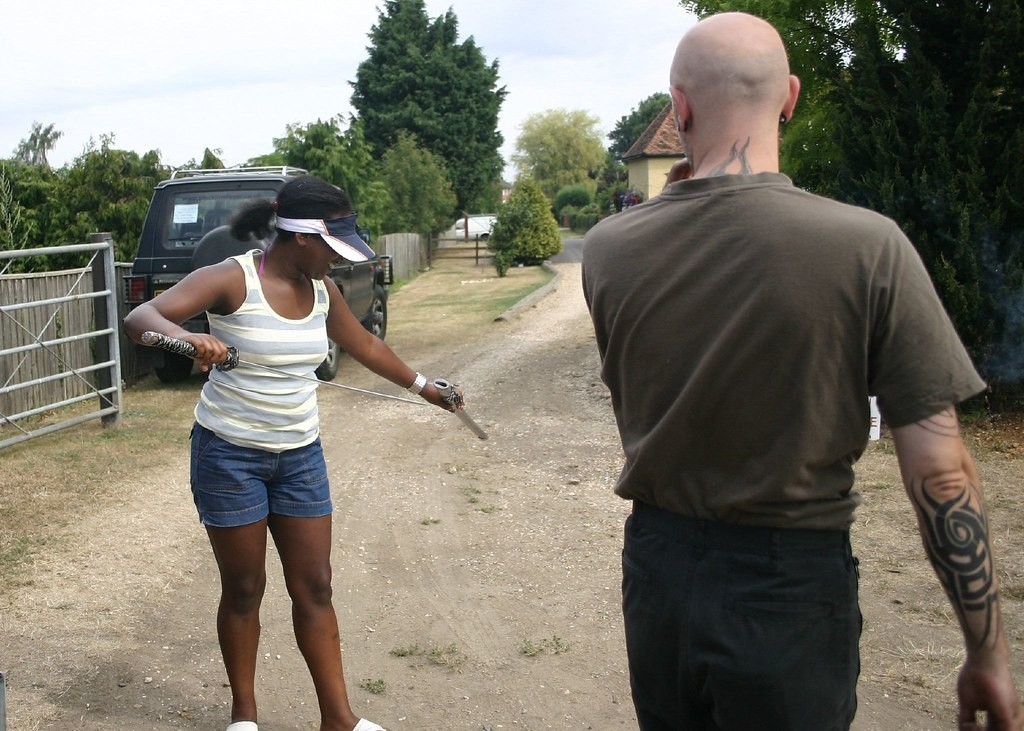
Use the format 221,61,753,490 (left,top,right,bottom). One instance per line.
274,212,376,263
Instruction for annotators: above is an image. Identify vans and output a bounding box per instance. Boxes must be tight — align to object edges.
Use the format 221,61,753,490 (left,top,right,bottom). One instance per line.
449,216,498,242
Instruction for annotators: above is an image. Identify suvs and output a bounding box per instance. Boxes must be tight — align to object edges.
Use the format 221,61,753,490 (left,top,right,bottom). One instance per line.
121,166,395,381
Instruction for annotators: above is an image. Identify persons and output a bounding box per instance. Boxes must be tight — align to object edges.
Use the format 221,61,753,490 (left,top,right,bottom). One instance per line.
123,176,463,731
582,13,1019,731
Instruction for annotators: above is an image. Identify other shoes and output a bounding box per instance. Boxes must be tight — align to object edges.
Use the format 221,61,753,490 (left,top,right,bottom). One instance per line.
226,721,258,731
353,717,386,731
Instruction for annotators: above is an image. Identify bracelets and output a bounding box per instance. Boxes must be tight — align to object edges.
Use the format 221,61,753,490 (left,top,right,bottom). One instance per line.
408,372,426,394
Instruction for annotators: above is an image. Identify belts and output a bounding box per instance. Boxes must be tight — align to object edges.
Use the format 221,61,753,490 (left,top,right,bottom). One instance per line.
631,501,848,555
